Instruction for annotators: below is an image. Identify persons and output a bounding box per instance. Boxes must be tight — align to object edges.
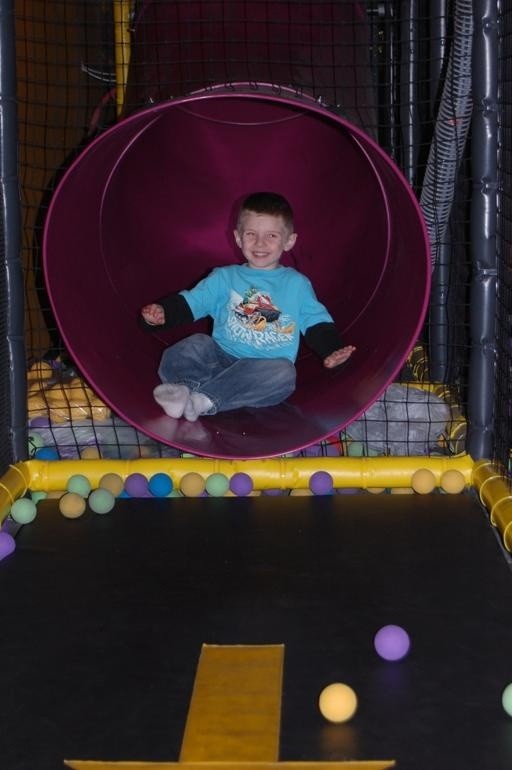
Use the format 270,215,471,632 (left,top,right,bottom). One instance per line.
138,190,356,422
